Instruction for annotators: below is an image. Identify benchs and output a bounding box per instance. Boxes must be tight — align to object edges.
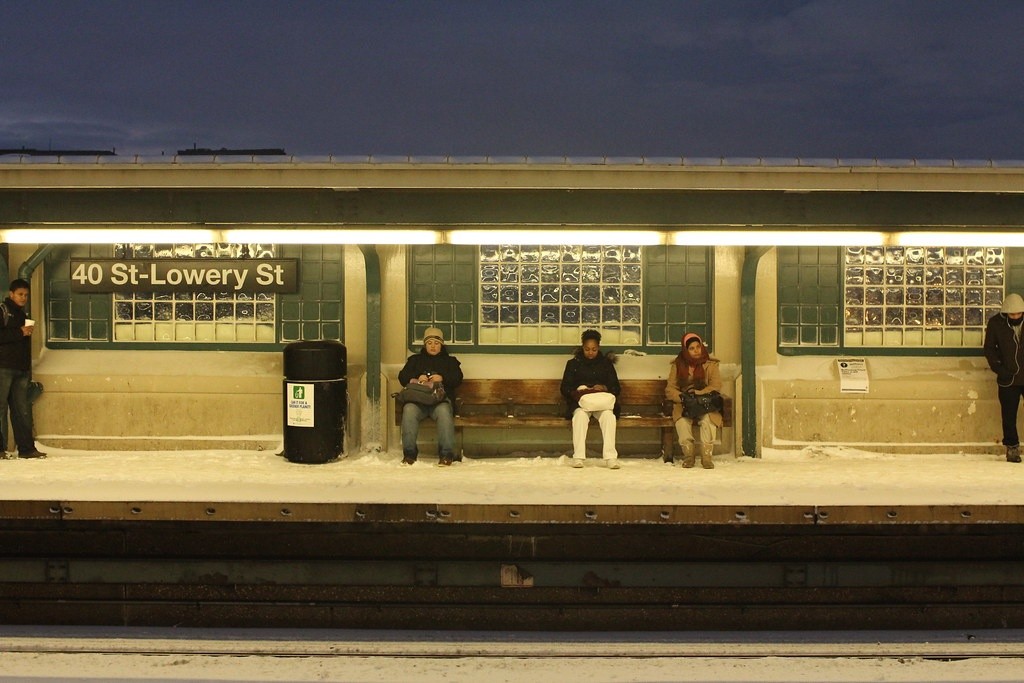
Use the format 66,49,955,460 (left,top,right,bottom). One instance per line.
396,378,733,464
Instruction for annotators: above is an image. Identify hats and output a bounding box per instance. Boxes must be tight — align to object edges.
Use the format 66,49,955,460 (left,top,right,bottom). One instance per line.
423,327,444,346
684,333,702,348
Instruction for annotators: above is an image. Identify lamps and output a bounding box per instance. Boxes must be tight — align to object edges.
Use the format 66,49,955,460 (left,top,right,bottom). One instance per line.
0,222,1024,248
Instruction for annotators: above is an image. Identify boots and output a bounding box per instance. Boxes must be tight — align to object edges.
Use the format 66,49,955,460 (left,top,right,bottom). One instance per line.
682,443,695,468
700,444,714,469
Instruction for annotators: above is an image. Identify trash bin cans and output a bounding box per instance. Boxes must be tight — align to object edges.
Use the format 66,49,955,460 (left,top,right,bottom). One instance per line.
280,340,349,464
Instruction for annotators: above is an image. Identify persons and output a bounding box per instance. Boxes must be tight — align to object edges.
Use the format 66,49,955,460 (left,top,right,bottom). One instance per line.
983,293,1024,463
0,279,46,458
665,332,724,469
397,327,463,467
560,330,621,469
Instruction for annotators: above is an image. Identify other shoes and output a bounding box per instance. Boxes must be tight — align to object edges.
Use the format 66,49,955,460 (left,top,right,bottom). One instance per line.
573,459,584,467
606,459,620,469
18,449,48,459
1006,448,1021,462
437,456,452,467
401,457,415,465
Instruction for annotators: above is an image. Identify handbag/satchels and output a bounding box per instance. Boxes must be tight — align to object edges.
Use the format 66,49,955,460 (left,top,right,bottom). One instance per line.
679,390,723,418
577,385,616,411
391,381,446,405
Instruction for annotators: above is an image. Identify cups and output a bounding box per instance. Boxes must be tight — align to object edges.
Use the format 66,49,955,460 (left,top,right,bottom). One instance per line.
25,318,35,329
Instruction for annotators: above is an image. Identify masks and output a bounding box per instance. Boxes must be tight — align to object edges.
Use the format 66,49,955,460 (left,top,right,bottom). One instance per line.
1009,314,1023,326
